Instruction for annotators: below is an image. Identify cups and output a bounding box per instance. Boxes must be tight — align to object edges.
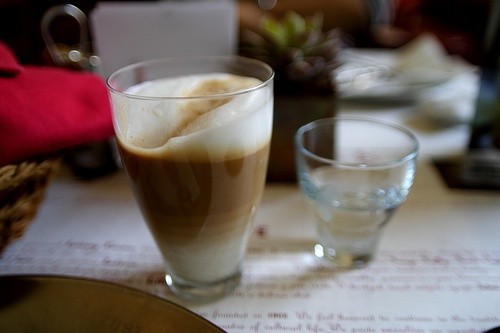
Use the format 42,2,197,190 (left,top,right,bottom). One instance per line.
294,116,421,266
106,55,275,299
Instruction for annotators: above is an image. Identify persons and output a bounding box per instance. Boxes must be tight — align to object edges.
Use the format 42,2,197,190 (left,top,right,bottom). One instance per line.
327,0,475,58
